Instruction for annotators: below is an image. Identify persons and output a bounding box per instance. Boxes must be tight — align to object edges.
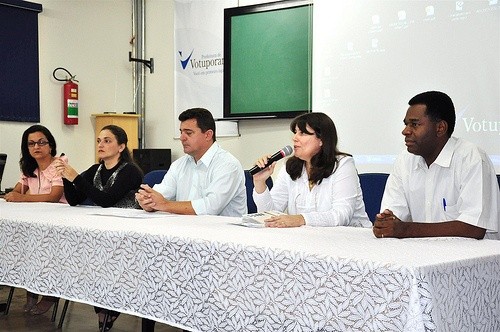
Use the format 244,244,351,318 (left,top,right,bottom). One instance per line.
135,108,249,220
373,91,500,244
54,125,144,332
2,124,74,317
251,111,373,231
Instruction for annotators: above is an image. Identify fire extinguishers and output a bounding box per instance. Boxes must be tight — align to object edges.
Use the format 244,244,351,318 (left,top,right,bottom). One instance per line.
53,67,79,125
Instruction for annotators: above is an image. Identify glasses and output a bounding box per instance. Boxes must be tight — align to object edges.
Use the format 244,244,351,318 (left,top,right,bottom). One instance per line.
26,141,49,146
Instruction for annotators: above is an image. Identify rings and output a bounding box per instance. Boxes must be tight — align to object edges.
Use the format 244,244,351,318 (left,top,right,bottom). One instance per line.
382,234,384,238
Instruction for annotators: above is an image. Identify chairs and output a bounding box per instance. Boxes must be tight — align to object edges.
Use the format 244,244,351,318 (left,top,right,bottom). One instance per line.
359,173,391,225
242,169,274,215
0,199,500,332
2,284,60,322
57,170,168,330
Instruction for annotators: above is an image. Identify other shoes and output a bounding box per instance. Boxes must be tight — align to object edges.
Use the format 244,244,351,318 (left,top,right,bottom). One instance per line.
22,298,37,312
30,299,54,315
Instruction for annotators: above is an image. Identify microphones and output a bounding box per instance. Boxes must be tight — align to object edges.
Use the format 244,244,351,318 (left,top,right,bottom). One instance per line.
249,144,292,175
